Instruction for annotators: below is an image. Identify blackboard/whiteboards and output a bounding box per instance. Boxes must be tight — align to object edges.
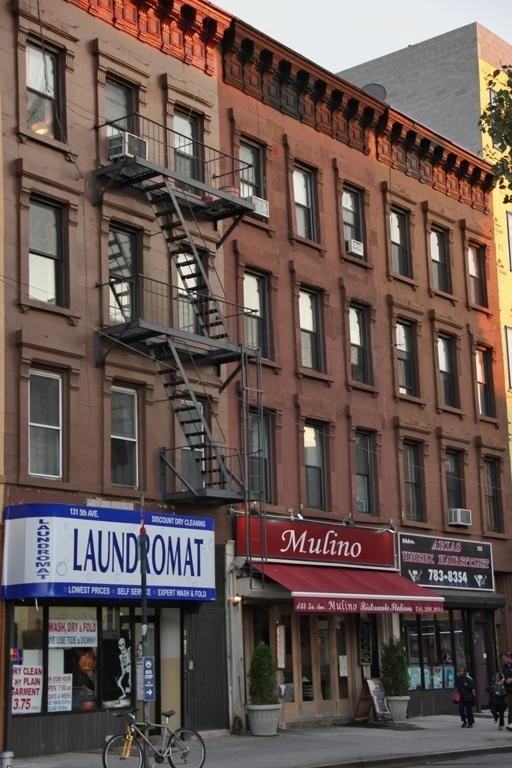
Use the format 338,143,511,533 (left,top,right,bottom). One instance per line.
365,677,392,722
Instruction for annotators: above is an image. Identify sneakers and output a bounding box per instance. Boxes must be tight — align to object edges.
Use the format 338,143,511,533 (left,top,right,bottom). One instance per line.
461,718,512,730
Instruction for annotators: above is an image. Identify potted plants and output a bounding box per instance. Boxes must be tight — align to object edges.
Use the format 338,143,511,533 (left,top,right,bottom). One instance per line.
245,641,283,737
380,638,411,721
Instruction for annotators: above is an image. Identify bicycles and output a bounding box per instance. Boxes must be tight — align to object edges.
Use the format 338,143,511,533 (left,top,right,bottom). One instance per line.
101,706,207,768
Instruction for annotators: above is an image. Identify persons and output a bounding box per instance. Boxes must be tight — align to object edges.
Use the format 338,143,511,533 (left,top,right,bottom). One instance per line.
454,665,477,728
64,646,96,694
499,651,512,732
485,671,510,730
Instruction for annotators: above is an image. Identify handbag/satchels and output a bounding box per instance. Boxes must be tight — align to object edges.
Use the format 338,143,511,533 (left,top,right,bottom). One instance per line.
452,691,461,703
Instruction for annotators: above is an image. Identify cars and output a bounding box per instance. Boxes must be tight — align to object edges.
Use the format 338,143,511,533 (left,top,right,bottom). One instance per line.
102,689,131,709
68,685,97,711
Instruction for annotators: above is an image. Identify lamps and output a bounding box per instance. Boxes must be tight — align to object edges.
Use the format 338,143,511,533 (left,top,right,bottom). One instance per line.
230,594,242,606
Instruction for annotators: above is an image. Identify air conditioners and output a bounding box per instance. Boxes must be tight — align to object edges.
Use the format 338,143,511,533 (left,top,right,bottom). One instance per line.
449,508,472,526
246,196,269,219
107,133,148,162
345,239,364,257
